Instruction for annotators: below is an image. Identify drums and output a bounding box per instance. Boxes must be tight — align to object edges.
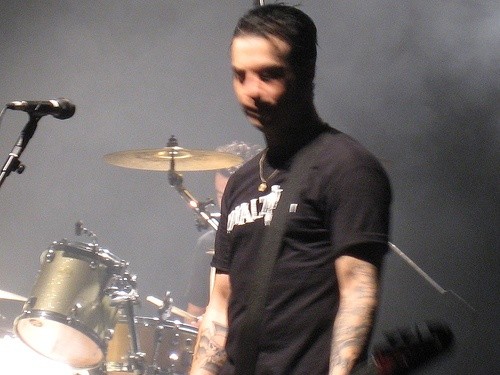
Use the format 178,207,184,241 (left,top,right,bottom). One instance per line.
105,314,199,375
13,237,143,372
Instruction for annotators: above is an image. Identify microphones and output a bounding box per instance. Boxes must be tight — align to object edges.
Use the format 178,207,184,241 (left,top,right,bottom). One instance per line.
6,98,75,120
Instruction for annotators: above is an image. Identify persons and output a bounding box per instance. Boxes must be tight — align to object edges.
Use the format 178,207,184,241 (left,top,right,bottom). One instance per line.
183,141,263,327
188,4,392,375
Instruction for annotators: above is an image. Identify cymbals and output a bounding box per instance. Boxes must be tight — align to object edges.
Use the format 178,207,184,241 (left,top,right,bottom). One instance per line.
0,290,29,303
99,146,245,172
